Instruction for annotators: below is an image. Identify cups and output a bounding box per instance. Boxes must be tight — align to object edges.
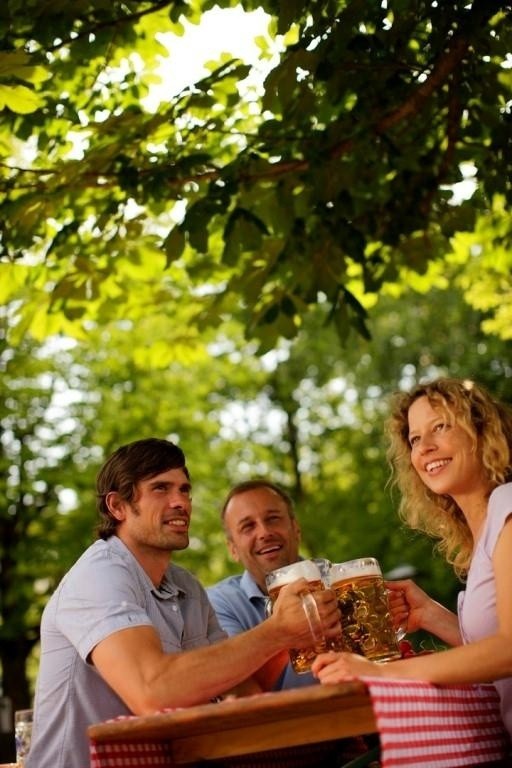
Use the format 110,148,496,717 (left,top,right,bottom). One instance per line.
262,559,348,675
265,558,330,619
329,557,408,665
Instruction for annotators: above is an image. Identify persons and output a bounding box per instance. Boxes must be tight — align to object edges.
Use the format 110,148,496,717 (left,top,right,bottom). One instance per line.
204,484,324,692
22,438,344,768
314,381,512,736
